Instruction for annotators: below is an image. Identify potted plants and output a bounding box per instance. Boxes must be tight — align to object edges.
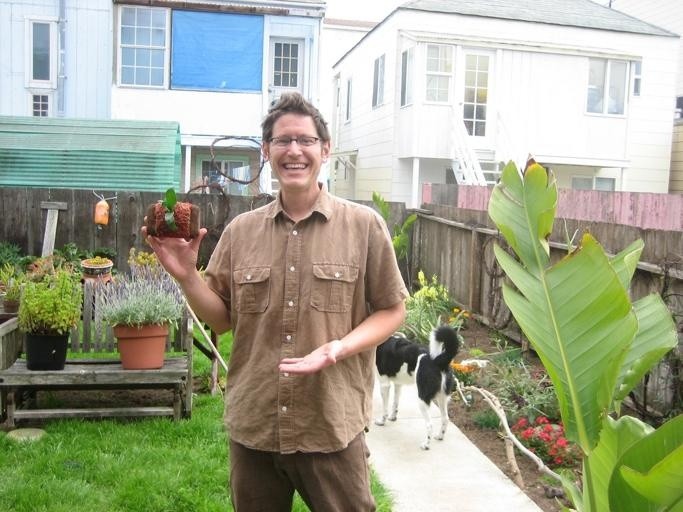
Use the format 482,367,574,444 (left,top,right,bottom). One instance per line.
2,256,184,370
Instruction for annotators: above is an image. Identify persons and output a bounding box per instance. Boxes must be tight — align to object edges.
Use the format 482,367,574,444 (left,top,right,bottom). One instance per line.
141,92,410,512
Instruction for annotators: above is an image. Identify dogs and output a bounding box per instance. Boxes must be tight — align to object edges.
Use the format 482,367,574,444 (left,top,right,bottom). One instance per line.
372,326,460,452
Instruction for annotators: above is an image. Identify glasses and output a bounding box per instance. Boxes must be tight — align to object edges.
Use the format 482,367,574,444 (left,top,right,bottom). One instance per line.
269,137,324,146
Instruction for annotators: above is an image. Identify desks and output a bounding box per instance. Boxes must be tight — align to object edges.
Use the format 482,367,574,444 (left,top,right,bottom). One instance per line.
0,358,191,430
